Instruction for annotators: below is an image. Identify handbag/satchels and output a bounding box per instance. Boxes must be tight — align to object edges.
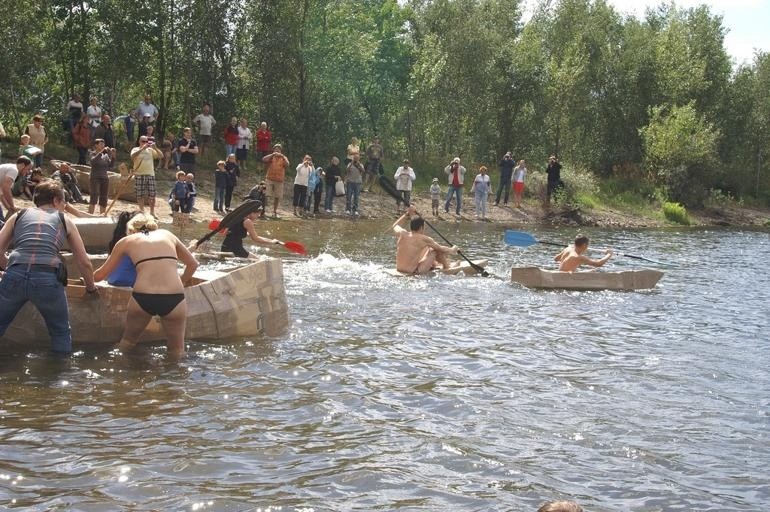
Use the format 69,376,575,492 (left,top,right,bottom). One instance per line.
55,263,68,286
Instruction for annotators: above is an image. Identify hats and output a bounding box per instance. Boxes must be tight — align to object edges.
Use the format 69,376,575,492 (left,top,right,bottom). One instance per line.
432,177,439,183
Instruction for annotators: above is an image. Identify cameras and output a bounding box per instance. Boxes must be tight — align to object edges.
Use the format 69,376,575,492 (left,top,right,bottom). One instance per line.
104,146,114,154
504,155,509,159
451,162,458,164
147,142,154,147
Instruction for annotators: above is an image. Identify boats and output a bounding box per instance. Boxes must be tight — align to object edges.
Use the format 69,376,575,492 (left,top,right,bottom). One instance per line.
49,159,148,204
512,265,664,292
194,250,237,261
384,260,492,279
0,257,290,345
63,216,119,248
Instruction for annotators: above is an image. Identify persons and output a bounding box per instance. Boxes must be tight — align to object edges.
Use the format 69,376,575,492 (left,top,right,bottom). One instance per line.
469,166,494,219
0,93,385,228
491,150,515,209
394,158,417,221
1,178,101,356
391,204,459,277
535,497,580,512
510,159,528,209
107,210,198,286
429,177,441,217
443,156,467,218
64,211,201,354
222,199,279,260
545,154,565,208
552,232,616,274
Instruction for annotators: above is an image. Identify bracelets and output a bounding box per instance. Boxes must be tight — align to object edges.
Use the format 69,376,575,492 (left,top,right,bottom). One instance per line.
80,277,85,285
85,285,97,292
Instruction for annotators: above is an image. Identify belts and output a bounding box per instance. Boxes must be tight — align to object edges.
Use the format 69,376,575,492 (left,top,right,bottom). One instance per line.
12,265,57,272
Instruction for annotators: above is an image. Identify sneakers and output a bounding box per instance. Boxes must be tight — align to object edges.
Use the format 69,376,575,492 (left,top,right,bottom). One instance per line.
294,209,359,218
213,208,232,211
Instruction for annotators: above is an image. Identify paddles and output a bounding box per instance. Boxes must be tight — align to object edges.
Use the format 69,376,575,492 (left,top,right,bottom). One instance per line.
197,200,262,247
208,219,307,255
104,156,145,218
504,230,679,267
378,176,489,277
